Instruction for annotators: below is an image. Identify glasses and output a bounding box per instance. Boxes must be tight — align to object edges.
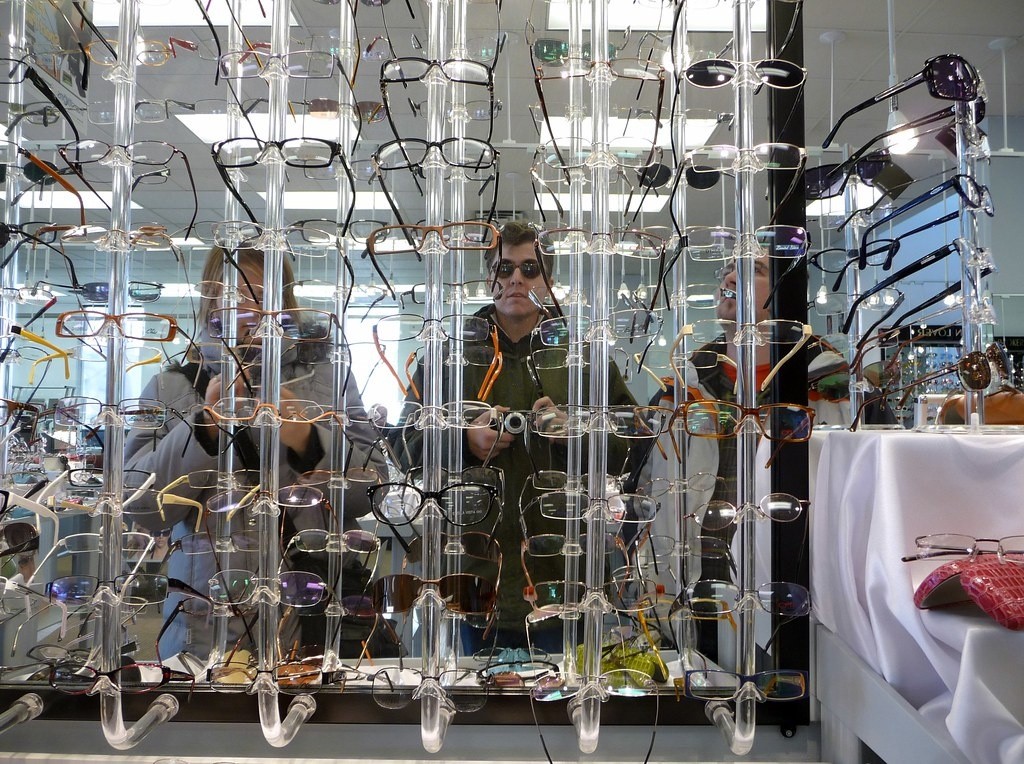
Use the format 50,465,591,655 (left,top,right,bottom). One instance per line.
0,0,1024,763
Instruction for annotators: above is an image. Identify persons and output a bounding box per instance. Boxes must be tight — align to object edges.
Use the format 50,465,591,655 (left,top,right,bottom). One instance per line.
367,404,394,437
124,233,390,658
386,223,650,657
618,246,900,681
